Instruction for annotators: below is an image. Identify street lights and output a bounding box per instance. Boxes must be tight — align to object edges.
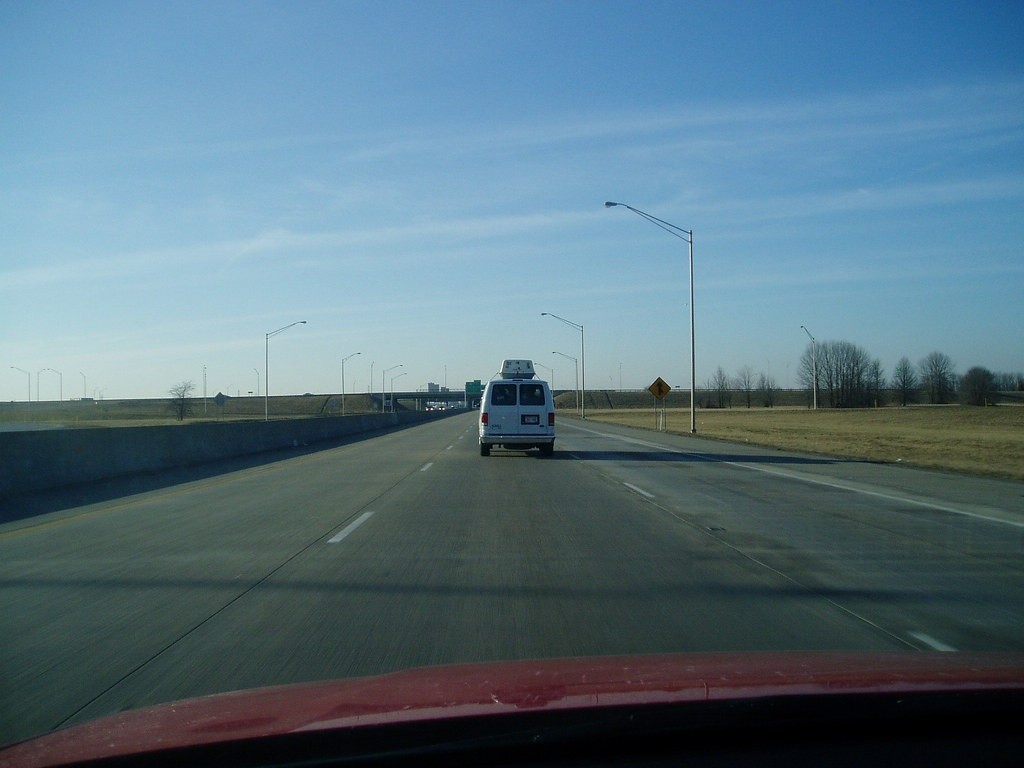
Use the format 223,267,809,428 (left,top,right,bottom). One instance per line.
390,371,408,413
552,351,579,415
382,363,403,413
254,367,260,396
370,359,375,396
264,318,308,422
619,362,622,393
603,199,698,435
801,325,818,409
202,365,207,414
541,312,585,420
340,352,361,416
9,365,90,401
534,362,555,410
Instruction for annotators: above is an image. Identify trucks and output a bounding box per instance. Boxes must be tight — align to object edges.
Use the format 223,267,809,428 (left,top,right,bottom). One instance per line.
479,357,557,456
